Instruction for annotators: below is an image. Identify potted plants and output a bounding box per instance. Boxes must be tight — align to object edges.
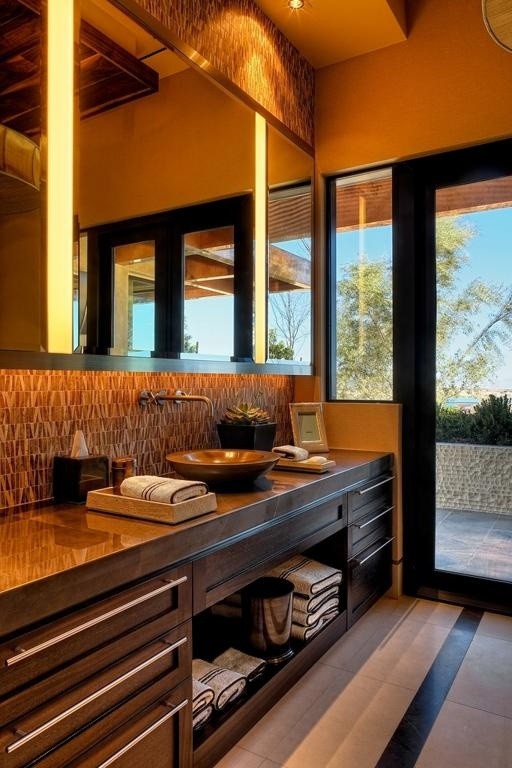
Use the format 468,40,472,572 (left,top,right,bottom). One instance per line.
216,397,277,452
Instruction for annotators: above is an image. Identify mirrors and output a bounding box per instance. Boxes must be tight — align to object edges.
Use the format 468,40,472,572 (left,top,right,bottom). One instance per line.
2,0,316,366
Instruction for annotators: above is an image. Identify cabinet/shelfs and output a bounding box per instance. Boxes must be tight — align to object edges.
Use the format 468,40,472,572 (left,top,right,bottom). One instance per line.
0,561,193,767
347,471,395,614
194,488,347,750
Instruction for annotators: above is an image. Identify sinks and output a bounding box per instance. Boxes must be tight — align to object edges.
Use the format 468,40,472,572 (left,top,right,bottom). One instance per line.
165,449,280,482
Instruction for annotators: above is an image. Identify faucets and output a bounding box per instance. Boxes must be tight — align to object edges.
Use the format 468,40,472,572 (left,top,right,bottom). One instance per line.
158,393,214,417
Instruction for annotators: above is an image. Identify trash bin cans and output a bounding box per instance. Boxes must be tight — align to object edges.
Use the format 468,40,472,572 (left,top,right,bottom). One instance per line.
240,576,295,665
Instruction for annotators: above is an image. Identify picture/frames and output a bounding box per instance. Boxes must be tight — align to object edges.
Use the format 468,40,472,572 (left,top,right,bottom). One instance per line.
288,400,329,454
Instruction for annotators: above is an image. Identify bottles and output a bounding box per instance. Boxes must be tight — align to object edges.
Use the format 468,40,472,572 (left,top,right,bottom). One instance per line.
111,456,137,496
